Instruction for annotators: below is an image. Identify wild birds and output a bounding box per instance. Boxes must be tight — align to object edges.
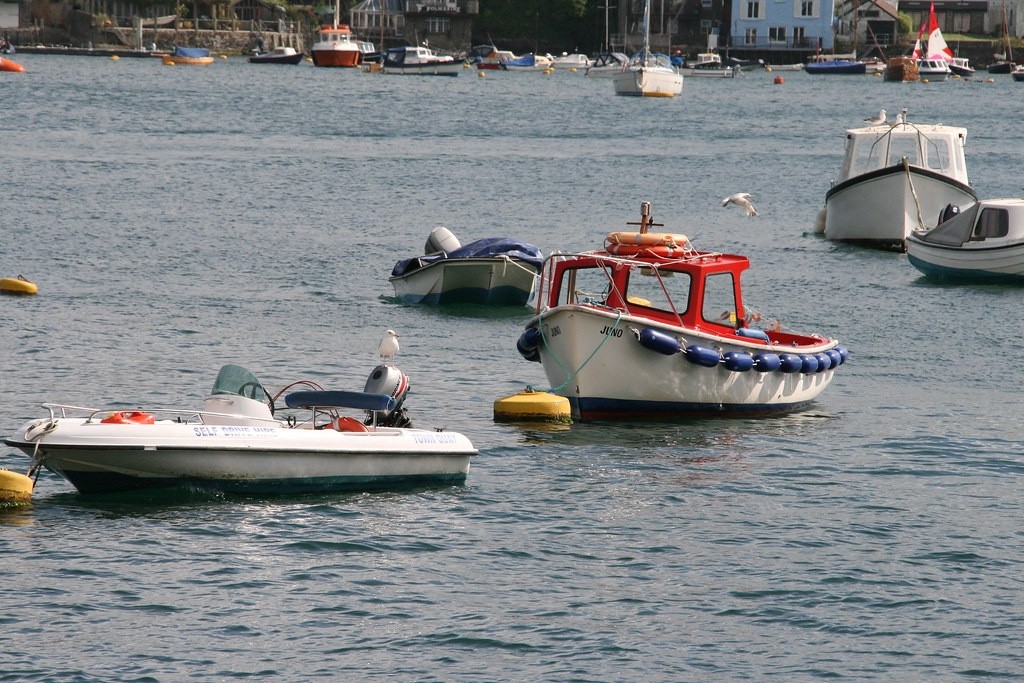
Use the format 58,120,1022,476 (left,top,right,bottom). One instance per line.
378,329,400,368
887,114,904,129
862,109,887,131
720,192,759,218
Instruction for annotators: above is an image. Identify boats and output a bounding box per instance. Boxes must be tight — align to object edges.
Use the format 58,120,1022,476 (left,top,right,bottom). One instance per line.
382,46,471,82
764,63,804,72
949,57,976,77
865,61,892,75
0,365,479,503
812,121,972,251
907,58,951,82
247,47,307,67
161,47,215,68
515,206,851,426
587,52,764,81
905,197,1024,284
388,226,549,308
470,44,553,72
804,60,866,76
311,0,388,66
554,54,599,71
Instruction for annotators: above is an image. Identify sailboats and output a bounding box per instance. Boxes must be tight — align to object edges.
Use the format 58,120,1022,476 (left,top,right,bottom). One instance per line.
612,1,684,98
986,0,1024,83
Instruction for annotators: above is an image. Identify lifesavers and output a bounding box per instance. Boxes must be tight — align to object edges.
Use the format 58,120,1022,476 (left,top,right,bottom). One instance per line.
325,417,370,432
606,230,688,257
